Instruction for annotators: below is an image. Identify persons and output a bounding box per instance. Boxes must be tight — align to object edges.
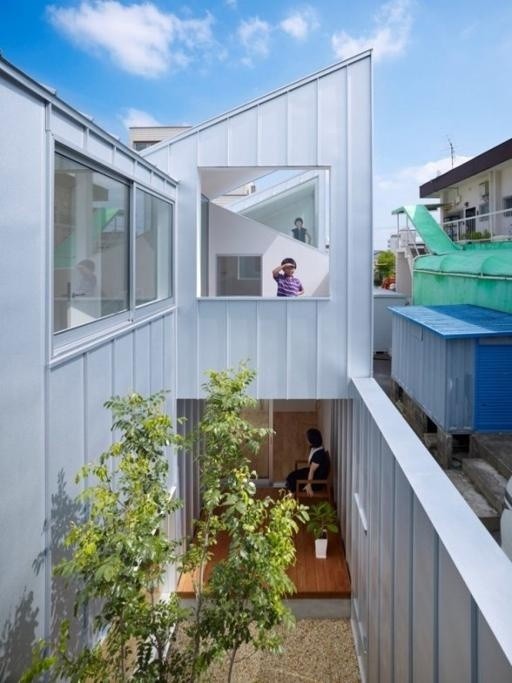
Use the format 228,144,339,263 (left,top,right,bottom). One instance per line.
271,257,305,296
290,216,312,243
285,426,330,500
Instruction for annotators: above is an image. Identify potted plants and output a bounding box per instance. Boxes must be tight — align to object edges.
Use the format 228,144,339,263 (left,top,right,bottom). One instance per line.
308,501,338,560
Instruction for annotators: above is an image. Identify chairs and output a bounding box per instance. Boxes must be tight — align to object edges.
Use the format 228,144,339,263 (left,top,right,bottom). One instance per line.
295,450,333,504
